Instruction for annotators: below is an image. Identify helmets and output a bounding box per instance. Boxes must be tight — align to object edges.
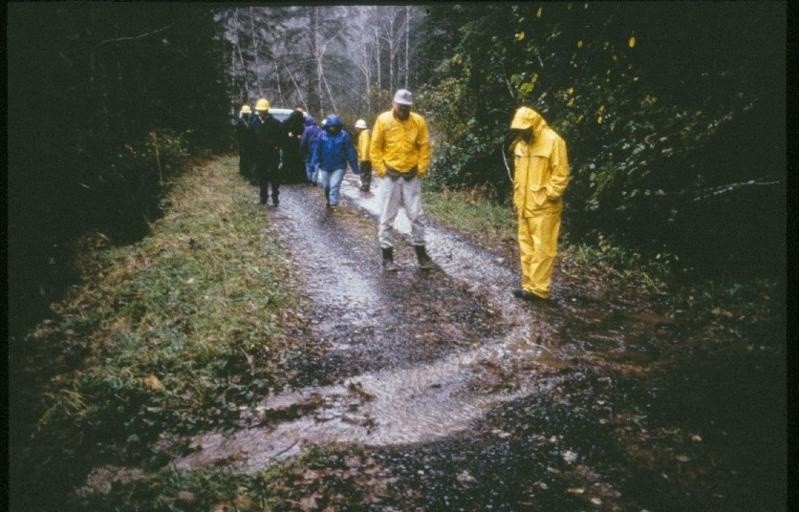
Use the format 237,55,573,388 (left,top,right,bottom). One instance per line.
395,89,413,105
240,105,251,113
254,99,270,110
355,119,367,129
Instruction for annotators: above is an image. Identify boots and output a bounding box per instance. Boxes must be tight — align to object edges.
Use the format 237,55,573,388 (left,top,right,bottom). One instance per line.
416,245,433,269
381,247,398,271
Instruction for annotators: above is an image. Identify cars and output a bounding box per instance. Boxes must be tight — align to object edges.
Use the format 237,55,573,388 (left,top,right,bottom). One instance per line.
261,107,314,180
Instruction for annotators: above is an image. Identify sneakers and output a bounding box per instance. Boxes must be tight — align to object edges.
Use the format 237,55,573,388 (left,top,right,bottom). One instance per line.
511,289,539,301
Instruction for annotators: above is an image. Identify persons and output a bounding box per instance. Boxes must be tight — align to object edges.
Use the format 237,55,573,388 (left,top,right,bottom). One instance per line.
234,97,372,213
369,89,434,270
509,107,569,302
